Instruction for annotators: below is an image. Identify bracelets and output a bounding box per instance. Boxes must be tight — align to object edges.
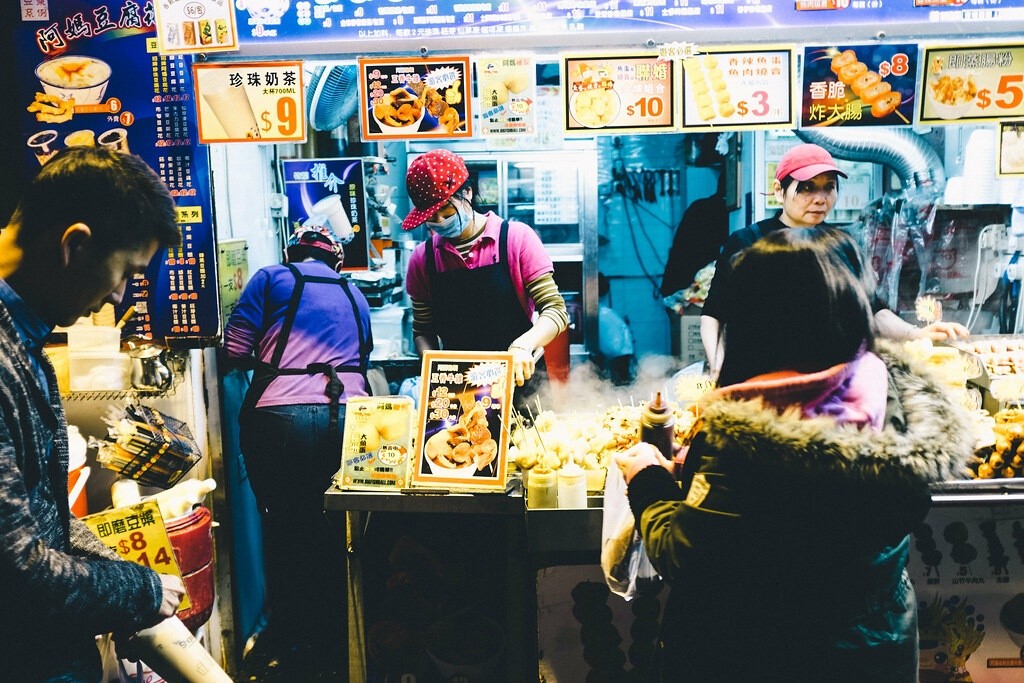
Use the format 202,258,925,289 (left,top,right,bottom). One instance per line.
507,342,532,354
906,325,918,339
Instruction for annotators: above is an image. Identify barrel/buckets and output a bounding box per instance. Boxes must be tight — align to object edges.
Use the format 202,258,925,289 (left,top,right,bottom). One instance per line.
107,495,214,629
67,424,89,520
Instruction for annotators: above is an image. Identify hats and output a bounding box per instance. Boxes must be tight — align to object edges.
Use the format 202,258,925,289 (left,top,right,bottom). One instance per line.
402,149,470,230
287,223,344,267
776,144,848,181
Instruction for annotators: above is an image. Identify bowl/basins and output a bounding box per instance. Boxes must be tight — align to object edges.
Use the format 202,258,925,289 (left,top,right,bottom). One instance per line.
372,94,426,133
35,55,113,107
424,429,477,476
928,71,978,120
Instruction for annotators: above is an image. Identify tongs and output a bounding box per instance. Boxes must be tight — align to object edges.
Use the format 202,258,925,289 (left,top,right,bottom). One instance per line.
514,346,545,387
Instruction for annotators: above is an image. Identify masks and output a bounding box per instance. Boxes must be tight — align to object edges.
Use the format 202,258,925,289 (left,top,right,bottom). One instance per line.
426,208,472,238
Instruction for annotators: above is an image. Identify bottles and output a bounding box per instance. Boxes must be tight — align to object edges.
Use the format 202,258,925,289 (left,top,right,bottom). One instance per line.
528,454,557,509
110,479,141,509
557,453,588,509
640,392,675,461
158,490,199,521
142,478,217,504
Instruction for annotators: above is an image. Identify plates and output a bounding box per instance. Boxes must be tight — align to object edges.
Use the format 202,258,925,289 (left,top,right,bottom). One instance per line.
569,87,621,129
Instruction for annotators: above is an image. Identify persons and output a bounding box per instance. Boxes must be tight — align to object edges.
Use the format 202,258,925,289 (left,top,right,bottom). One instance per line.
217,223,375,683
614,142,971,683
402,148,569,427
0,145,186,683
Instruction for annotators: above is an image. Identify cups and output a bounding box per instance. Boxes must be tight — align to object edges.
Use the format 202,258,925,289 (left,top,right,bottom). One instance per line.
64,130,96,148
26,129,59,168
97,128,132,156
202,75,261,139
